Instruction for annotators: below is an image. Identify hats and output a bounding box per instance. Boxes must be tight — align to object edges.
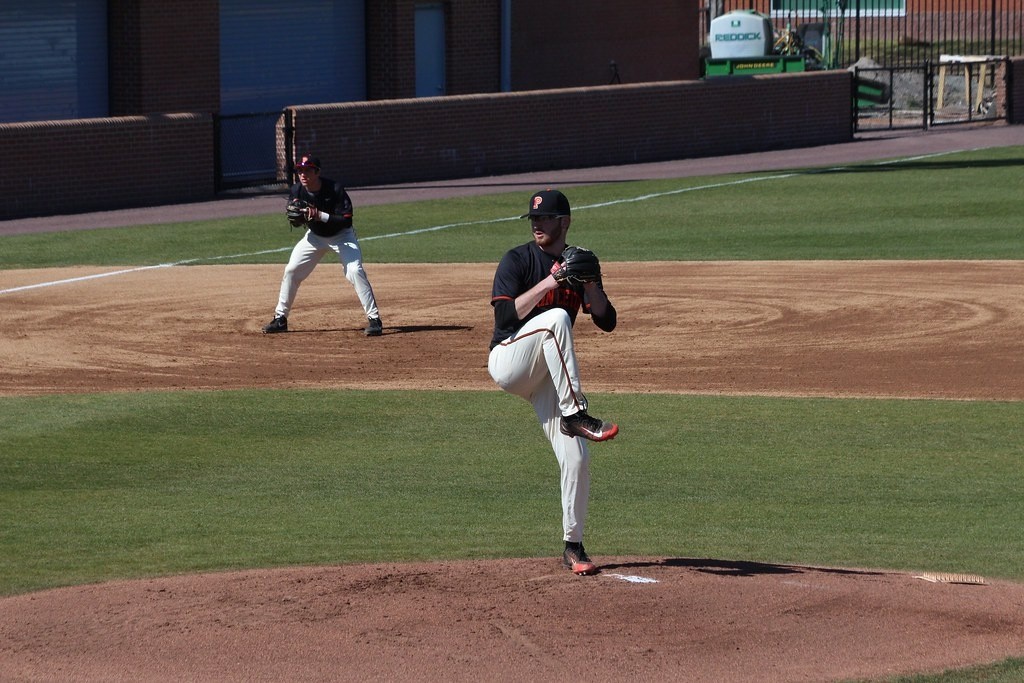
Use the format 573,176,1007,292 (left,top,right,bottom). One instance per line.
295,154,321,169
520,189,570,218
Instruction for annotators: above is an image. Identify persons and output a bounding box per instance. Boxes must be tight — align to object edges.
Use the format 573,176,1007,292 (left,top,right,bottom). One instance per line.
262,154,383,335
486,190,618,575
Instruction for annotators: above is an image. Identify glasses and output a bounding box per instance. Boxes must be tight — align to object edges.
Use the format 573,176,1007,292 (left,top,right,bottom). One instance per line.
528,215,569,223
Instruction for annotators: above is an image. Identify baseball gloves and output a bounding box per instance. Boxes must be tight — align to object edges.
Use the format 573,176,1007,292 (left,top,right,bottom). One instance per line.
550,246,602,288
285,198,320,228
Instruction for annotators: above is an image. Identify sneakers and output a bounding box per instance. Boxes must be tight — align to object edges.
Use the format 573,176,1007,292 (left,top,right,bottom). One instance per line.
562,546,596,576
262,314,287,331
364,316,382,335
560,410,619,442
